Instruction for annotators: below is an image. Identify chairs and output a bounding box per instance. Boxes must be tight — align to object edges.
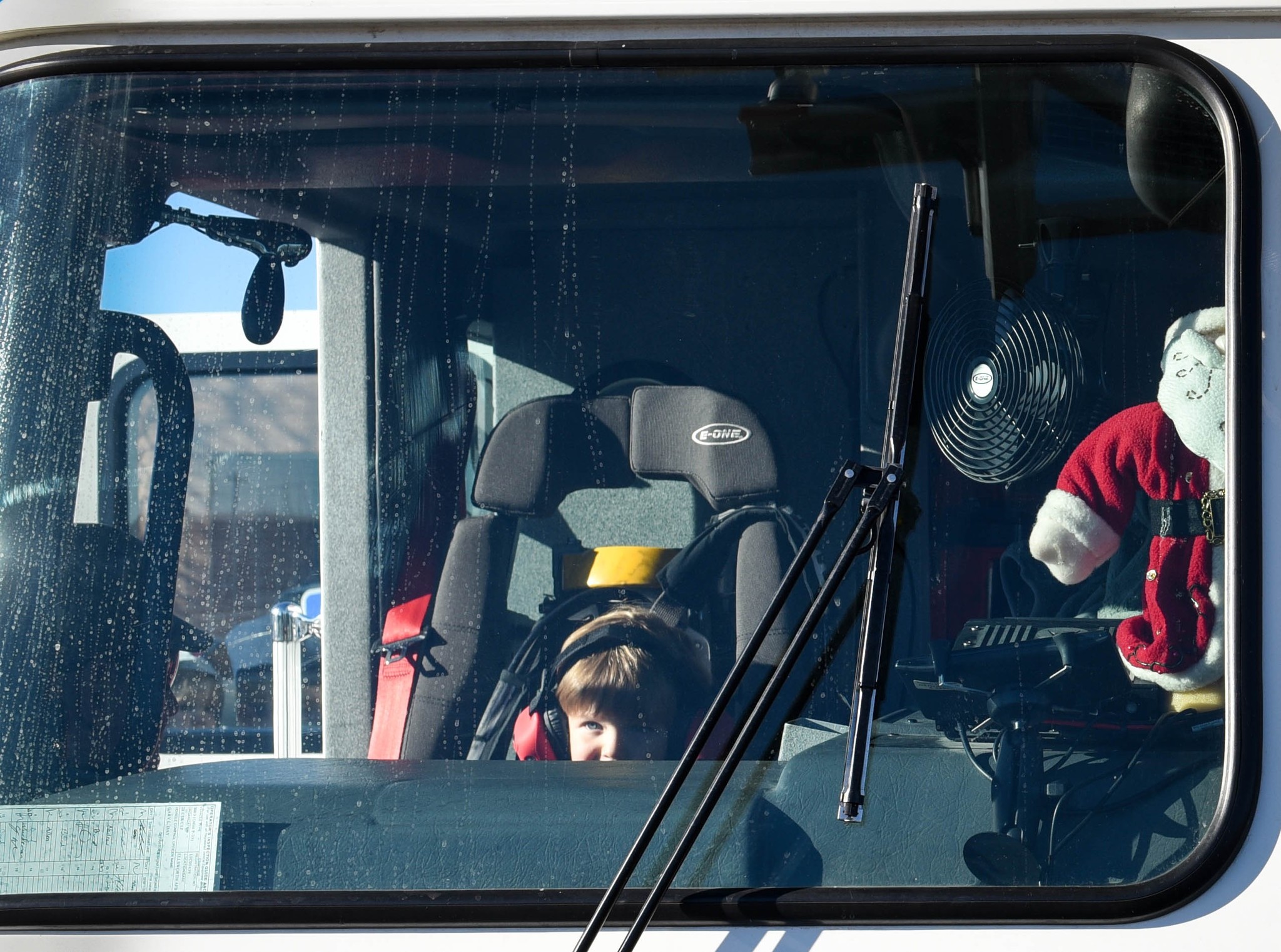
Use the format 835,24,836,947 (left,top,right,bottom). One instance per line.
400,379,804,761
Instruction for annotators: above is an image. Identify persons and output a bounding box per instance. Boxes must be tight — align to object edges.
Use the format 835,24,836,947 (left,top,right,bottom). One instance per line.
501,603,746,763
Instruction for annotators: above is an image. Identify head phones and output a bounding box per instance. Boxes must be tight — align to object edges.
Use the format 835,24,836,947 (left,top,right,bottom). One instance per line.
537,625,698,760
